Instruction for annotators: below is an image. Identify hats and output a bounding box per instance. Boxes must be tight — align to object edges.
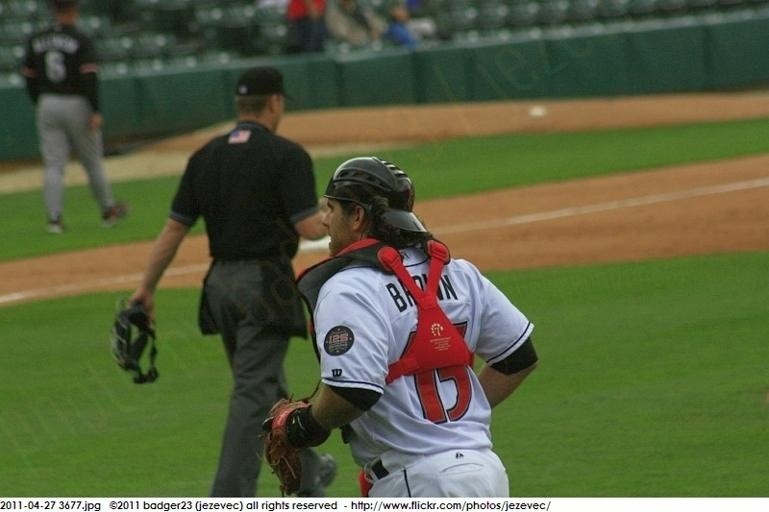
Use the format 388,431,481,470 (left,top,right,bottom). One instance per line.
235,67,291,99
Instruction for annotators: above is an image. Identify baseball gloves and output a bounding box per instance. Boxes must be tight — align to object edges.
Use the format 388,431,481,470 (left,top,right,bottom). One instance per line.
260,395,325,498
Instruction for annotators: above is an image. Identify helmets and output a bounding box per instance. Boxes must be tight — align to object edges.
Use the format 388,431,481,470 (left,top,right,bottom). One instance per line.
324,156,427,246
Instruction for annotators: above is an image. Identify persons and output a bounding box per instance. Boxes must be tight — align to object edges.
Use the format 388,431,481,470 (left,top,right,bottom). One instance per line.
267,155,541,497
246,1,438,57
21,2,133,235
124,63,330,497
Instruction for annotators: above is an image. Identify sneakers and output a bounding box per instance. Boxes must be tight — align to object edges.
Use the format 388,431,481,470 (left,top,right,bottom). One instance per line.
104,202,129,224
47,212,68,233
303,453,337,497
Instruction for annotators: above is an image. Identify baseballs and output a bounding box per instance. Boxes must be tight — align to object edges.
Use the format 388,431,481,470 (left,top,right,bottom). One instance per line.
529,106,546,117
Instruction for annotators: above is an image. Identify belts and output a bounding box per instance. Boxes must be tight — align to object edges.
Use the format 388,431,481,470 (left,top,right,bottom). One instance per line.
370,459,388,477
